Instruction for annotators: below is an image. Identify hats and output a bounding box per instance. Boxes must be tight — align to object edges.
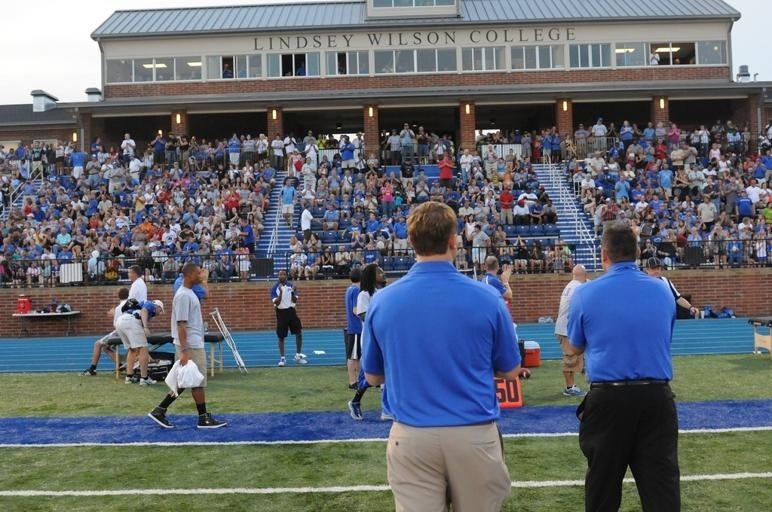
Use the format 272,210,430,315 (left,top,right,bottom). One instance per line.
646,257,663,269
151,300,165,313
254,185,261,192
518,200,524,204
598,186,603,190
91,154,97,157
605,198,611,201
356,131,364,135
539,185,544,190
702,193,710,199
59,217,65,222
364,191,373,196
505,186,511,190
26,213,34,218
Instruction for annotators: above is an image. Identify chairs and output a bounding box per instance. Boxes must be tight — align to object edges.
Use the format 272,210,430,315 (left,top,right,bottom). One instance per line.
504,225,517,237
324,232,336,245
395,255,410,269
314,232,322,244
531,223,542,236
543,222,558,237
383,256,394,270
312,207,326,218
517,224,530,237
340,218,351,229
512,189,539,200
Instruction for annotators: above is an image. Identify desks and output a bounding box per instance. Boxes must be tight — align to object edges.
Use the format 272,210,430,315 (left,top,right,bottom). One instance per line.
12,310,80,337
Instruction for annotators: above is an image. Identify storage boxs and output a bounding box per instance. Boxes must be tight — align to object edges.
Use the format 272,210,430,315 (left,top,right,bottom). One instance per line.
524,341,540,367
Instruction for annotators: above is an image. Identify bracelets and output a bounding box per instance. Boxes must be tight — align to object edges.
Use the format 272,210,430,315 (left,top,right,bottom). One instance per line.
144,327,149,329
689,305,694,310
179,348,189,352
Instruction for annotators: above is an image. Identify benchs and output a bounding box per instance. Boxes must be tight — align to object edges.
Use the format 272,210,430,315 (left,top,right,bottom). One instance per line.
109,328,224,379
748,316,772,355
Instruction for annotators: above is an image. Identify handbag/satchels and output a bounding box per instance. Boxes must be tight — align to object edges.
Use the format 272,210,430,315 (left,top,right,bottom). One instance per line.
294,160,302,171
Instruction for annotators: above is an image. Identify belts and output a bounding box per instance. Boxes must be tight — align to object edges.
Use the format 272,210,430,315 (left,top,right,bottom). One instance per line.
590,379,667,388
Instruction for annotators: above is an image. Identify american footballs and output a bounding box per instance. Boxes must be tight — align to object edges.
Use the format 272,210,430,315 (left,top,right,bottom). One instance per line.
519,368,530,378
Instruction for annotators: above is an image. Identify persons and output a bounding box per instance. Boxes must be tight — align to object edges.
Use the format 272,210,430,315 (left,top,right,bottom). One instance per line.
347,264,394,421
221,62,345,78
78,288,129,376
1,116,772,288
480,256,513,301
147,263,227,429
128,265,147,305
115,300,164,386
645,258,699,315
173,262,209,299
650,48,660,66
345,269,363,390
360,200,521,512
567,228,680,512
270,270,308,367
554,264,589,397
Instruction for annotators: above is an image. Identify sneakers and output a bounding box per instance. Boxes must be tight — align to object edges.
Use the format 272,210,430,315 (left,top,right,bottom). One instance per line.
381,413,394,420
348,400,364,421
348,382,358,389
197,412,227,429
296,359,308,364
125,375,140,384
140,376,157,385
277,359,286,367
79,369,96,376
147,406,174,429
562,384,587,396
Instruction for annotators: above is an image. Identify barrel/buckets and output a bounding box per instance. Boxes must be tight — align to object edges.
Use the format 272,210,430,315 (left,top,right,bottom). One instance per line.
18,295,32,314
